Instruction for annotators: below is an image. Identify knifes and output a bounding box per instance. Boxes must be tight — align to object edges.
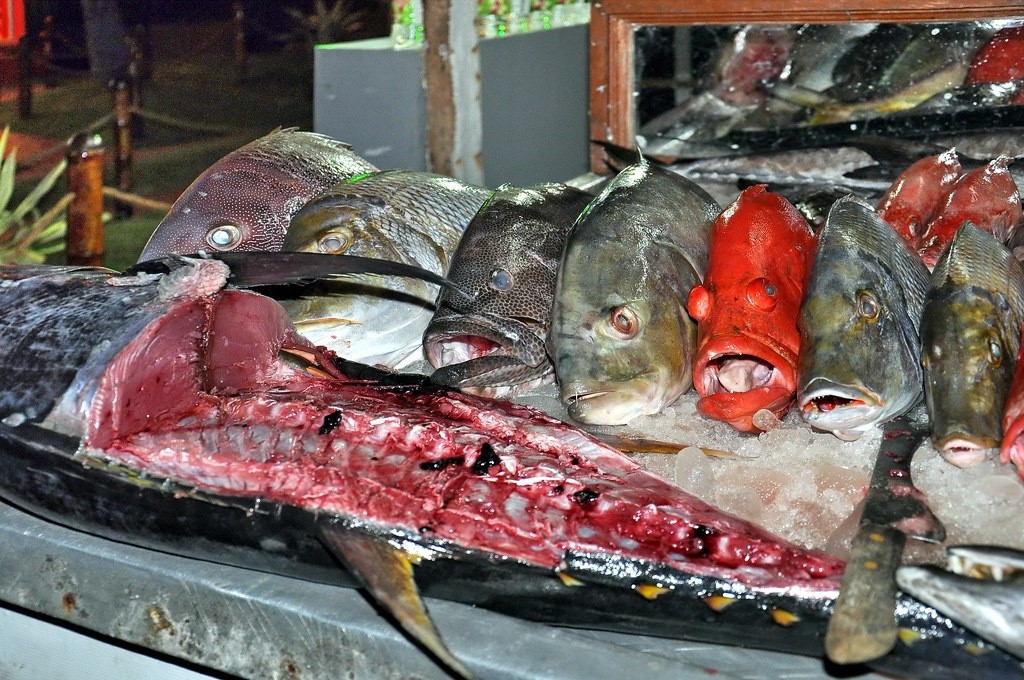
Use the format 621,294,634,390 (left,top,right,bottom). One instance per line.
823,416,949,665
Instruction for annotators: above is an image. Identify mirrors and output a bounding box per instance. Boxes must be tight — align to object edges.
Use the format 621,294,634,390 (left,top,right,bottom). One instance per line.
591,0,1022,225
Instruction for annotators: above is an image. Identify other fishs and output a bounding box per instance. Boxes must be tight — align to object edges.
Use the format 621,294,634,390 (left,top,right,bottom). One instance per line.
2,25,1017,680
